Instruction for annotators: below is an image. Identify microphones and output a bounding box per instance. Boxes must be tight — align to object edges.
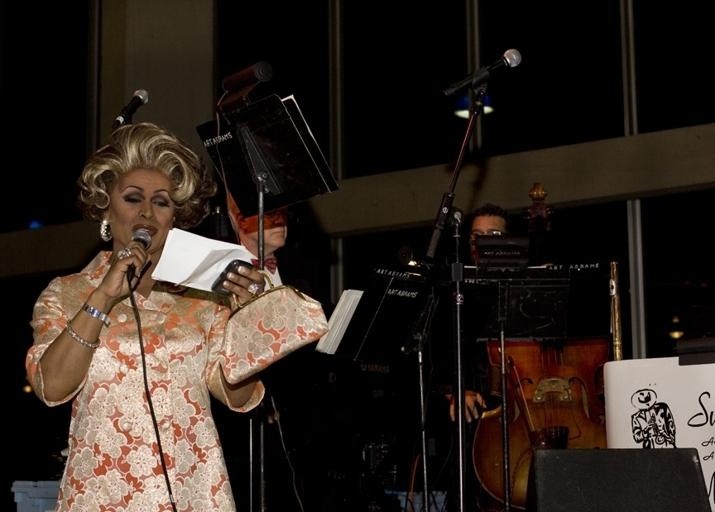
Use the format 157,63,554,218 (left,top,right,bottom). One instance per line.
126,228,152,280
443,49,521,95
111,88,149,127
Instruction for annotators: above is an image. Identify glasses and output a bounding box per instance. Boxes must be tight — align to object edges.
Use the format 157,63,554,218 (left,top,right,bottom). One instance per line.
469,228,507,241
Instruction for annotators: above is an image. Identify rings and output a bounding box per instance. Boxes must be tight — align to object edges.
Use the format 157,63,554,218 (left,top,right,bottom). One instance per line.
248,283,260,296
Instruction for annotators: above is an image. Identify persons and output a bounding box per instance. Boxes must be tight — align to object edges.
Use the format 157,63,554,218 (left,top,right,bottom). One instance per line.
25,121,266,512
227,189,297,292
446,204,512,424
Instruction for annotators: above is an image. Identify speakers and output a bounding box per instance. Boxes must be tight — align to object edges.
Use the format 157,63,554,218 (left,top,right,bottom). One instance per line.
526,449,711,512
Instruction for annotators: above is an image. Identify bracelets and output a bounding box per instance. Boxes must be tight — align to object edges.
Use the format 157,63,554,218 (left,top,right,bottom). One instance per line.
67,319,101,349
82,302,111,326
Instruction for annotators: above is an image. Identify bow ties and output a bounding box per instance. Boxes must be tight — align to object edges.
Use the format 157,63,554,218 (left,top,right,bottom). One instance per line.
250,256,278,275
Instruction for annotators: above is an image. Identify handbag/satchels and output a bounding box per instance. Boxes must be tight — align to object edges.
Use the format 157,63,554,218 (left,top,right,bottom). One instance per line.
215,268,333,387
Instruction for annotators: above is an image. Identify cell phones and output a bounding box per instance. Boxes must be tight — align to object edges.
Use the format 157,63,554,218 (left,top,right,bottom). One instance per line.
211,259,253,296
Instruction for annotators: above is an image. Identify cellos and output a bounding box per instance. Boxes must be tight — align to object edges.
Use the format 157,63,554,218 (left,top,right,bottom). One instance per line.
472,182,612,510
